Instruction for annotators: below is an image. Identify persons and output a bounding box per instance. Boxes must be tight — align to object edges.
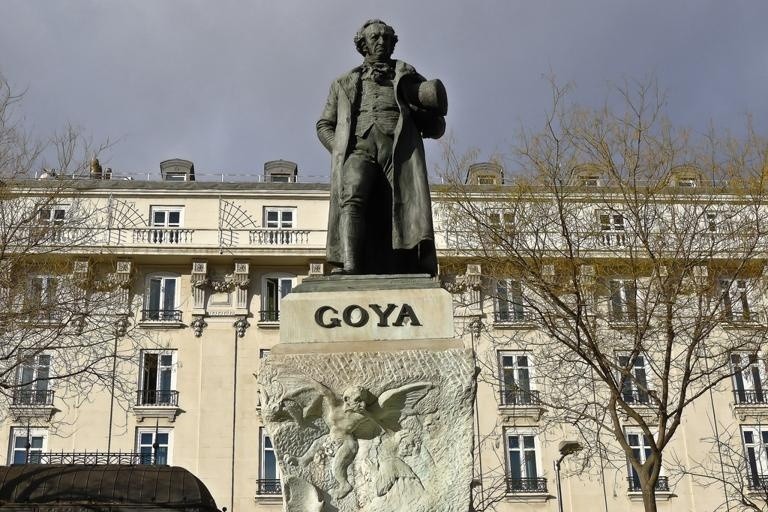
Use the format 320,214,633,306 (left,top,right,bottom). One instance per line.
279,382,433,500
315,17,449,277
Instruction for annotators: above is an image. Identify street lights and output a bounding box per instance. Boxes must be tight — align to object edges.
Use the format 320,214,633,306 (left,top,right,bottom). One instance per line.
554,438,582,512
